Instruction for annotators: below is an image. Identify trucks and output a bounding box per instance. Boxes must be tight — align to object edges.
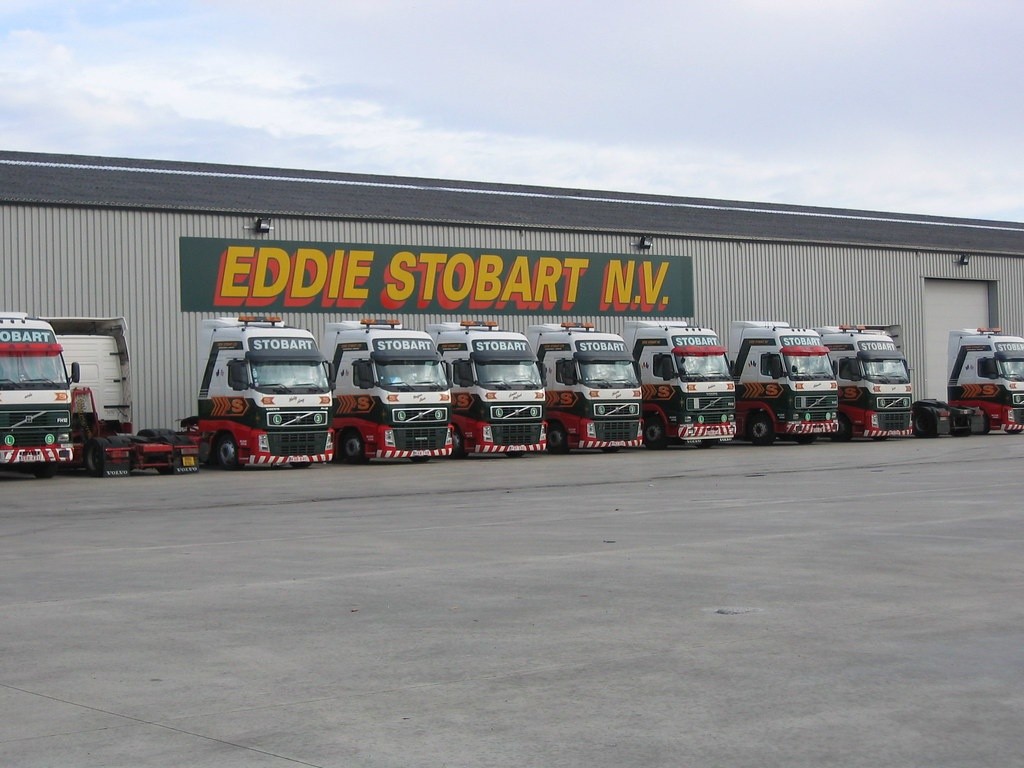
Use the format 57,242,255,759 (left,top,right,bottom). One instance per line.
424,319,548,460
0,311,80,479
54,333,200,478
312,318,455,467
815,324,913,443
527,323,643,456
623,320,737,451
135,315,336,471
911,327,1024,437
728,319,840,446
